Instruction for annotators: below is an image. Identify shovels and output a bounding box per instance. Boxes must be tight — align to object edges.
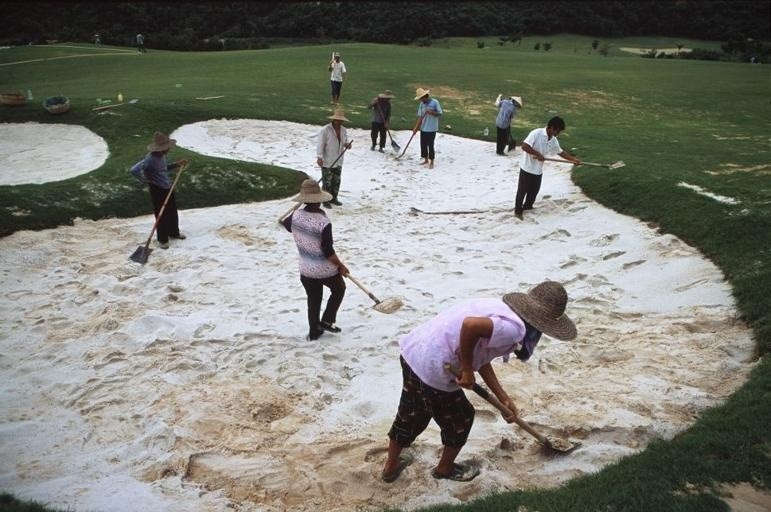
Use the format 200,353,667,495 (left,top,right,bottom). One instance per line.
345,272,402,314
376,101,401,153
500,96,516,152
91,98,138,112
443,361,582,453
531,156,626,171
393,114,427,159
129,165,185,265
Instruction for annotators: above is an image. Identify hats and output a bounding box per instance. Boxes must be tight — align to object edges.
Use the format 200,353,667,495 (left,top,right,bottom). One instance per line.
414,88,429,100
291,179,333,204
503,281,577,341
509,96,522,108
334,51,340,57
378,90,396,99
147,131,177,152
327,111,350,121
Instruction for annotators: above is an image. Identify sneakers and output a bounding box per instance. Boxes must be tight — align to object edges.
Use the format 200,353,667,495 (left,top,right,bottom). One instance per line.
317,321,342,333
175,234,186,239
323,203,331,209
159,241,169,249
382,452,414,483
431,462,480,482
330,201,342,205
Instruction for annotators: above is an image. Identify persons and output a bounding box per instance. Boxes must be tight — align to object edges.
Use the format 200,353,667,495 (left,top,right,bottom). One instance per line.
380,280,579,483
413,87,443,168
316,107,352,209
514,116,581,217
494,93,523,156
136,31,148,53
282,178,351,342
128,131,189,250
327,52,346,105
367,89,397,153
